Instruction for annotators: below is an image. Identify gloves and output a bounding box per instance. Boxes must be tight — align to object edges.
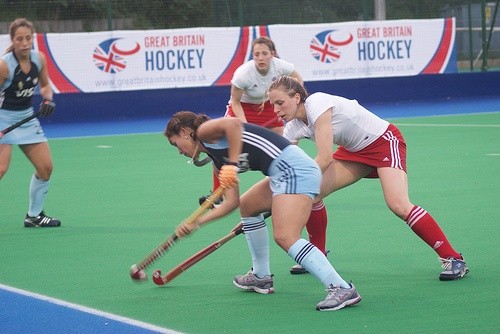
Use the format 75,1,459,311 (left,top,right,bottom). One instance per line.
219,157,238,189
39,100,56,117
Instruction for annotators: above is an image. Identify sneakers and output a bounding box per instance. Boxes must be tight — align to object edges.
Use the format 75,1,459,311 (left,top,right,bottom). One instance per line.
233,267,274,293
199,191,223,209
290,250,329,273
316,281,361,312
436,254,469,281
24,210,60,226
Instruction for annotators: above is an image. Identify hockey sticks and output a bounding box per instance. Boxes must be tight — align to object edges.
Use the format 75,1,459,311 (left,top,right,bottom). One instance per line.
0,110,40,138
150,227,249,284
127,183,228,280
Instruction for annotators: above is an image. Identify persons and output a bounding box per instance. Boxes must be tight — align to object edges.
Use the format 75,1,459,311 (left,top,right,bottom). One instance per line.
0,18,63,227
199,37,304,209
268,75,470,281
164,110,362,312
